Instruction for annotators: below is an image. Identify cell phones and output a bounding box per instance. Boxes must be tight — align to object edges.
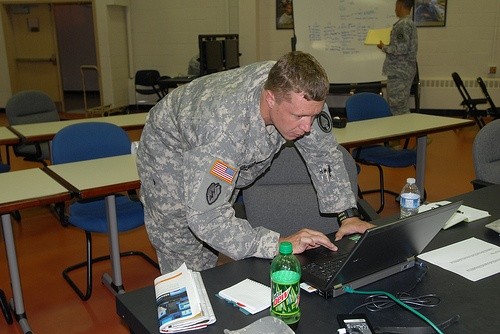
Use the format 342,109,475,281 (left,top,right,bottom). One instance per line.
337,313,375,334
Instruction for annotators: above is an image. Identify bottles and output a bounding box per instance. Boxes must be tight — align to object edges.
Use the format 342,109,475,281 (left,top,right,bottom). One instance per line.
400,178,421,219
268,242,302,330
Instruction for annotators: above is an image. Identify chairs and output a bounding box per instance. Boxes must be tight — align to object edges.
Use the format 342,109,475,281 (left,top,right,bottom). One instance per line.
348,93,427,215
471,119,500,190
451,72,487,130
6,92,83,227
477,77,500,120
134,70,161,112
52,122,160,300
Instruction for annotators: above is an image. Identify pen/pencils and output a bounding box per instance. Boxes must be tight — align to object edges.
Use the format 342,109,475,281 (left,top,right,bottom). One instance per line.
435,203,464,215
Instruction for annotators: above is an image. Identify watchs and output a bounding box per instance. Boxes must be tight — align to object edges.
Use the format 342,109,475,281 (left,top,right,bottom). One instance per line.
338,207,359,222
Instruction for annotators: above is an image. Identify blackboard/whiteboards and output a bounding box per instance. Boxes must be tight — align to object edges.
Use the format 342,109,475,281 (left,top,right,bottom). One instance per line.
291,1,414,85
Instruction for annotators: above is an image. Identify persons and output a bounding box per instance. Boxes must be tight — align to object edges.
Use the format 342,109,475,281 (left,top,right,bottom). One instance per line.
135,50,378,276
279,5,292,24
377,0,418,145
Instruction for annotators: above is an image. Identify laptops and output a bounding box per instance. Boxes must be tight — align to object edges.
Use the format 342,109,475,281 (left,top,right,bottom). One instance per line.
270,199,463,298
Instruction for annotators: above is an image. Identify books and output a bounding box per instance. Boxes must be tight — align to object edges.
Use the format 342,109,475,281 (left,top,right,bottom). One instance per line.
417,200,492,230
154,261,217,333
219,278,271,314
364,28,391,46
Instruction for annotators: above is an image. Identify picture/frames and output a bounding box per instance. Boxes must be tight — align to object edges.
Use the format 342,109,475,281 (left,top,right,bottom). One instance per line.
276,0,294,30
412,0,447,27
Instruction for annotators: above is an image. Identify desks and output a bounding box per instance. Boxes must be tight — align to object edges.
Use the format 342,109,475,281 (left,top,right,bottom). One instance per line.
43,113,473,303
9,113,147,142
0,168,71,334
158,78,194,95
117,184,500,334
0,126,18,145
